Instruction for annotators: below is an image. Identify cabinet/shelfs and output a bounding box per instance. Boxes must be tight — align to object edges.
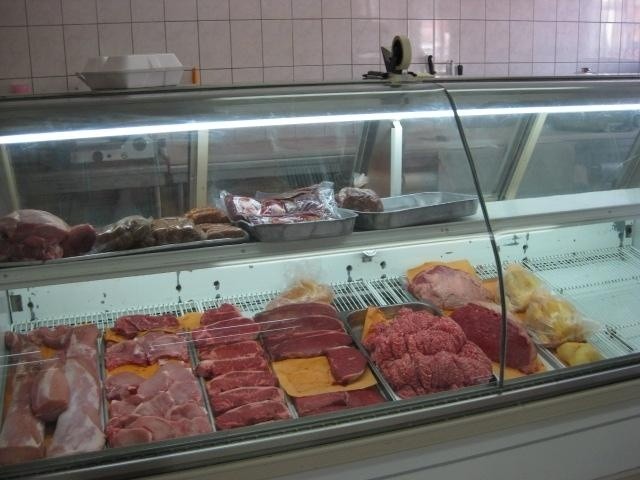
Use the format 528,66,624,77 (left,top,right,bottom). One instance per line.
0,65,640,479
